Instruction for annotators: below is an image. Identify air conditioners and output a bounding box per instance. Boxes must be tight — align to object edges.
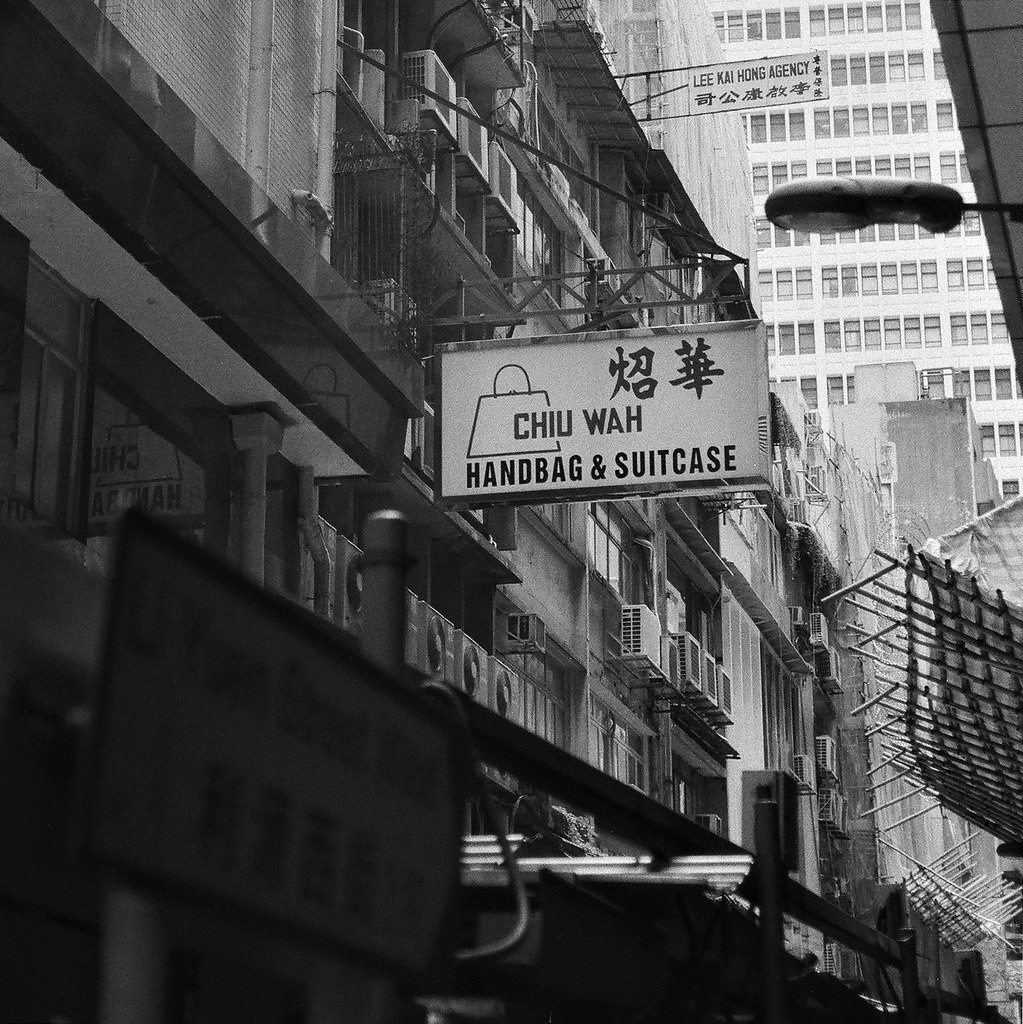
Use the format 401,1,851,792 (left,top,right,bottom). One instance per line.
486,141,520,233
804,409,821,431
816,645,842,688
361,278,419,353
788,606,807,627
400,49,457,144
792,754,815,790
694,812,722,834
508,613,545,652
818,787,848,834
585,256,619,299
620,604,735,726
446,98,490,188
808,467,826,496
809,611,828,649
824,943,842,980
816,735,837,780
